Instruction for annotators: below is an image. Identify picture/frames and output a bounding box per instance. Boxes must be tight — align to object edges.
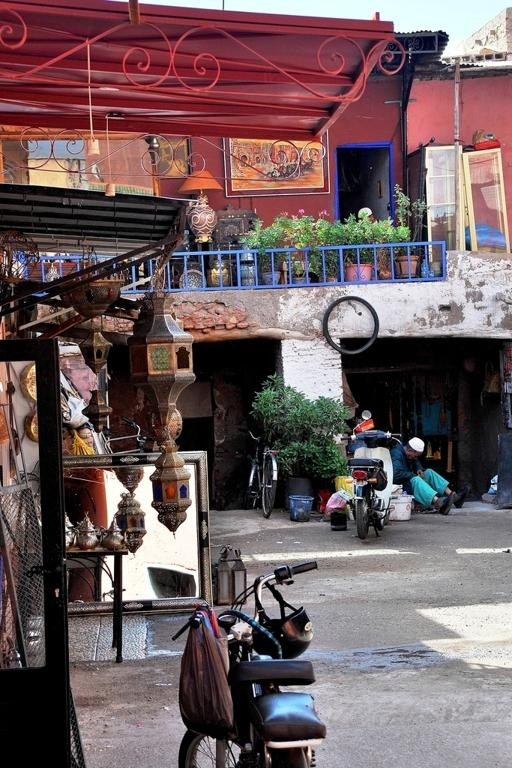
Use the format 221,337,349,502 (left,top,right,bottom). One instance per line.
156,135,193,179
222,129,331,198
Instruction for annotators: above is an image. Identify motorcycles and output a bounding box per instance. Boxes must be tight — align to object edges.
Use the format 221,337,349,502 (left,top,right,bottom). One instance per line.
172,559,327,767
342,411,405,542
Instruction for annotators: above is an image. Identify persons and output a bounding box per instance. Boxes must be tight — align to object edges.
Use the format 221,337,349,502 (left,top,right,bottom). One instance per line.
356,207,379,225
389,435,467,516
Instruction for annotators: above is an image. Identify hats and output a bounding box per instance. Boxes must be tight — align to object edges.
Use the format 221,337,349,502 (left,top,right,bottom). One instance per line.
408,437,425,453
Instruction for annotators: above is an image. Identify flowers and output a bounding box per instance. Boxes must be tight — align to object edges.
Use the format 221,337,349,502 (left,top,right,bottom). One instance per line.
279,208,329,277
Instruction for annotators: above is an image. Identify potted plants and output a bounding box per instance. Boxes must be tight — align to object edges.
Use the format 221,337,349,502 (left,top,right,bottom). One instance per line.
238,217,282,285
327,182,426,286
249,371,352,515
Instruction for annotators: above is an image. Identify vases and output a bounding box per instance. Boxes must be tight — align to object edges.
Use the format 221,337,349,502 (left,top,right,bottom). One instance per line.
293,277,312,284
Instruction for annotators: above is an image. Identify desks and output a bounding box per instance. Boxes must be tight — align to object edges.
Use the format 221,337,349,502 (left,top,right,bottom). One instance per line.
12,549,128,663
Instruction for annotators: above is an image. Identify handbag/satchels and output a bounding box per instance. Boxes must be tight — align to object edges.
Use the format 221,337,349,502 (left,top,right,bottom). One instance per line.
179,611,234,741
373,465,387,490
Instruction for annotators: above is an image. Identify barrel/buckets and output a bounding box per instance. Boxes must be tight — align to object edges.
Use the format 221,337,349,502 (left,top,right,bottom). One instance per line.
288,495,314,522
304,454,347,531
389,494,413,521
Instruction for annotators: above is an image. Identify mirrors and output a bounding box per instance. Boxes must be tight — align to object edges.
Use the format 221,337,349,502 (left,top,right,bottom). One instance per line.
62,449,213,616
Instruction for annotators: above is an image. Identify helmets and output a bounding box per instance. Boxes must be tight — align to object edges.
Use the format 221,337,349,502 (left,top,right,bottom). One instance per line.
254,606,313,659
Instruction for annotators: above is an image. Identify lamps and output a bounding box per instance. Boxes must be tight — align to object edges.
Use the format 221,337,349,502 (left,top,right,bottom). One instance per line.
177,170,225,244
83,35,116,198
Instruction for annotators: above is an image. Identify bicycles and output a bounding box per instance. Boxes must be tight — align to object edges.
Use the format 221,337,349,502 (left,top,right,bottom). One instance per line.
248,431,281,520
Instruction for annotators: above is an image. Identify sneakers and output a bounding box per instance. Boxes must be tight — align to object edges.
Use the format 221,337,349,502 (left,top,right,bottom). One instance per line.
453,487,469,508
439,492,455,514
